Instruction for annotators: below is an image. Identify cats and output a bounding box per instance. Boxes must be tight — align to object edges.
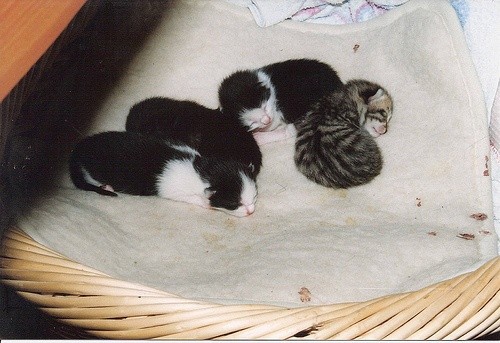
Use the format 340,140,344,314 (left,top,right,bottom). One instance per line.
67,95,262,217
217,59,393,187
